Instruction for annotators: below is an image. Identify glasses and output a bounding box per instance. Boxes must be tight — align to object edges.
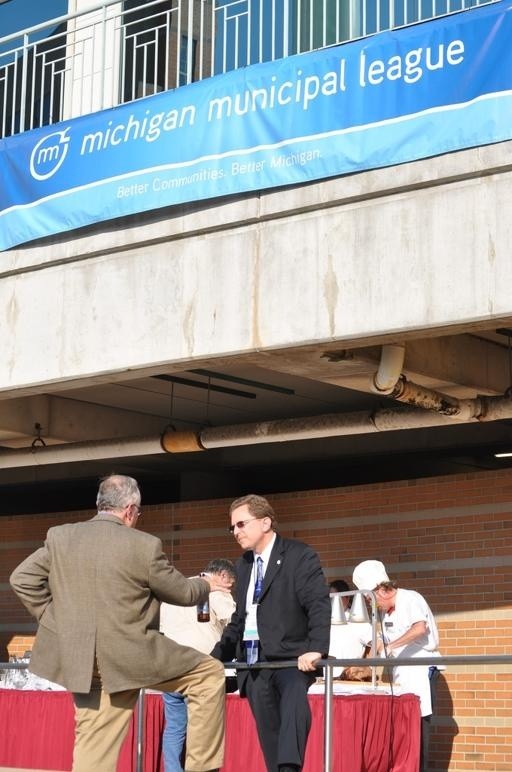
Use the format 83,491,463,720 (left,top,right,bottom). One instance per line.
229,517,254,533
127,503,143,516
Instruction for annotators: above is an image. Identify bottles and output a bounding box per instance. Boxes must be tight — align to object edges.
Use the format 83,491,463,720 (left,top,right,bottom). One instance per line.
196,572,211,623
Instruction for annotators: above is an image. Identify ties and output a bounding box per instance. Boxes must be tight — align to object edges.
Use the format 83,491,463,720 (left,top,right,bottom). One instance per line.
243,557,264,666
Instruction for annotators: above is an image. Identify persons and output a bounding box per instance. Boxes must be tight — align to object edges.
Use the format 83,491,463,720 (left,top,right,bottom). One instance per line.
343,580,447,771
208,493,335,772
320,578,382,684
156,556,237,771
6,473,233,771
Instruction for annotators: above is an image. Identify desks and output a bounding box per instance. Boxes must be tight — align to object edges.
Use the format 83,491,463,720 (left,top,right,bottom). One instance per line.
0,688,423,771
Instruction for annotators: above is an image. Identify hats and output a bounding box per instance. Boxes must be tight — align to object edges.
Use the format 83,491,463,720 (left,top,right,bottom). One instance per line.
352,560,389,596
